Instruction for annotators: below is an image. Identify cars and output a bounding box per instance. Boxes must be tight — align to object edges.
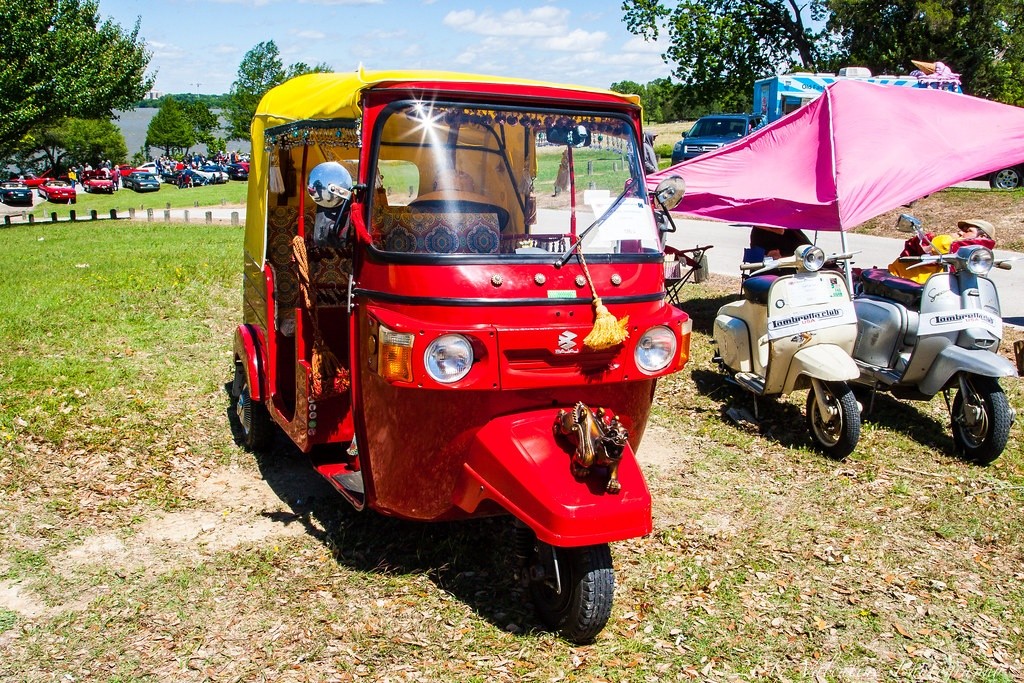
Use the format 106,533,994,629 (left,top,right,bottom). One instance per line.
122,171,161,192
672,112,769,166
58,169,118,194
110,164,149,178
37,178,76,202
137,153,250,186
0,181,33,204
9,168,57,188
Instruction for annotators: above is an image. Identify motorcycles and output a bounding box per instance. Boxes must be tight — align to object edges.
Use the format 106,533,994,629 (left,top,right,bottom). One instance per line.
838,215,1018,467
710,242,864,459
231,62,695,646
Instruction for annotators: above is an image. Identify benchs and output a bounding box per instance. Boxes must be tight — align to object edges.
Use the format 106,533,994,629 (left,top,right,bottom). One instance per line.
268,205,564,373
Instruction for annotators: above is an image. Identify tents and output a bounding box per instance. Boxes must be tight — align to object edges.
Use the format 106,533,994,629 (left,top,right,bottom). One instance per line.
623,80,1024,302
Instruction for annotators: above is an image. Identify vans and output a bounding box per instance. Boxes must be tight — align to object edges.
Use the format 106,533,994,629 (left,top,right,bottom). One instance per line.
751,65,1024,193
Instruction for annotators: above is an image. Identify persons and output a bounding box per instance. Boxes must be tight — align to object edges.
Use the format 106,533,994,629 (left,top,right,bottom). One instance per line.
641,131,664,175
19,148,251,191
744,123,754,137
746,222,813,276
955,219,995,239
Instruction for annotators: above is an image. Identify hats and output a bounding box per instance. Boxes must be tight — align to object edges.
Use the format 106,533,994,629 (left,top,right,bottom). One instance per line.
652,131,658,136
958,219,995,240
407,169,510,232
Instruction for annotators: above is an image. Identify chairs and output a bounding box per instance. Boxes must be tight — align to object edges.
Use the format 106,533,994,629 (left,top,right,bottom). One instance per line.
664,244,714,309
